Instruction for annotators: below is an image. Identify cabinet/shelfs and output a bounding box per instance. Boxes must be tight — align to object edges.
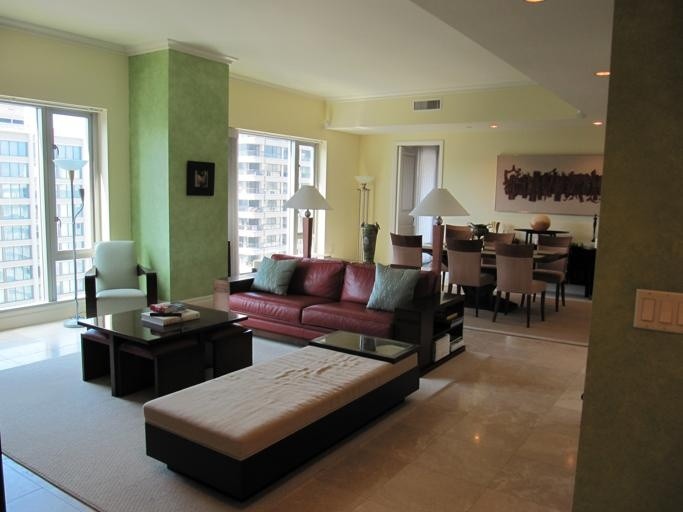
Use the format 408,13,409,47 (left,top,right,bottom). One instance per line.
434,291,466,362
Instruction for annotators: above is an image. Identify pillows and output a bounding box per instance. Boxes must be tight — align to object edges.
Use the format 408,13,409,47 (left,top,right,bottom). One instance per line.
366,259,421,313
252,253,301,296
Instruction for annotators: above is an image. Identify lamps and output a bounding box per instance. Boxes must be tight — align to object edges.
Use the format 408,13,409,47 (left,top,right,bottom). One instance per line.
51,157,95,330
282,183,334,260
353,174,375,262
407,184,470,295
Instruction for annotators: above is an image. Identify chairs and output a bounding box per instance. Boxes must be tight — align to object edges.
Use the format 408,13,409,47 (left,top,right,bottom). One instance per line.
387,232,423,269
421,225,572,331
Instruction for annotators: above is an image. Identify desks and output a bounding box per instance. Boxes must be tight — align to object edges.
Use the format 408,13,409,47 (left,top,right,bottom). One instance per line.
514,239,598,299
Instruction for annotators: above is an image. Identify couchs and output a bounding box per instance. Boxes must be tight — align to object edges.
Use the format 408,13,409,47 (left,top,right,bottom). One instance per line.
84,240,158,319
210,252,439,377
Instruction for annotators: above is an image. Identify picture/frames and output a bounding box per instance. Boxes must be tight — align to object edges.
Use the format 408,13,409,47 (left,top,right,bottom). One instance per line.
184,159,215,198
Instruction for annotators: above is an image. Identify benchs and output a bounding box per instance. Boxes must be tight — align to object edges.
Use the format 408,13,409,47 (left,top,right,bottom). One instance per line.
142,326,422,508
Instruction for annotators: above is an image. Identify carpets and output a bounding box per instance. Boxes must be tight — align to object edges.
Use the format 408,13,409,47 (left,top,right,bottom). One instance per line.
1,349,585,511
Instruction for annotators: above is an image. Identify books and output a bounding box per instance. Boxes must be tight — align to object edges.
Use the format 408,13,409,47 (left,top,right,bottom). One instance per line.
139,308,199,326
148,302,185,313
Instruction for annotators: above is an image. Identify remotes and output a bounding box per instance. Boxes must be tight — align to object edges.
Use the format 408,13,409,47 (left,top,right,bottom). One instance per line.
151,312,181,317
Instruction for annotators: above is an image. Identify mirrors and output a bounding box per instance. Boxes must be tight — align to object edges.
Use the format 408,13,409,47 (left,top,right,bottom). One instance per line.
309,329,417,366
